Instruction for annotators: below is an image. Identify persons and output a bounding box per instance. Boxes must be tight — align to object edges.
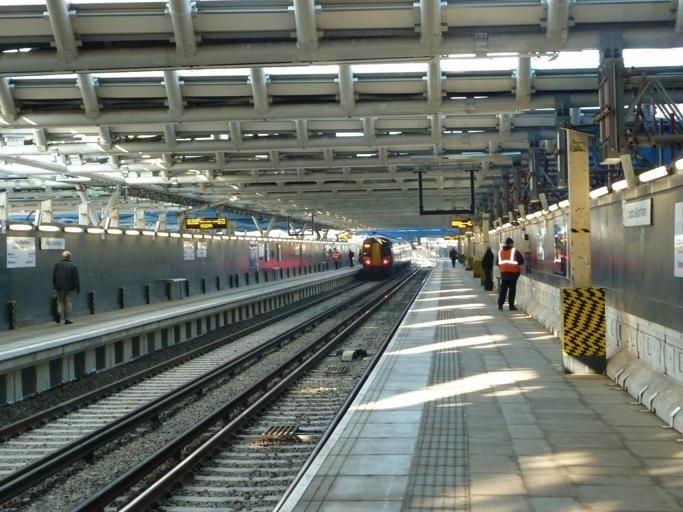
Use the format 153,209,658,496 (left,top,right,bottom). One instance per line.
332,248,340,269
349,249,353,266
450,247,457,267
52,251,80,324
482,248,494,290
496,237,524,311
359,250,362,265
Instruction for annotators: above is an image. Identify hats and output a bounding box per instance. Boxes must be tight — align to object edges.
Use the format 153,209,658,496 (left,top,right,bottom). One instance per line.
506,238,513,244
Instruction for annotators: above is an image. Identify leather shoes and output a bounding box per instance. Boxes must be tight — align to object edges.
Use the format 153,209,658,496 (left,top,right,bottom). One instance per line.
509,305,517,310
65,320,72,324
56,313,61,323
499,305,502,309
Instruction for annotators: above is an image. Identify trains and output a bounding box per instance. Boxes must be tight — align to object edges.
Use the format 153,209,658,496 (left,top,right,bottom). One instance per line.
362,236,412,279
